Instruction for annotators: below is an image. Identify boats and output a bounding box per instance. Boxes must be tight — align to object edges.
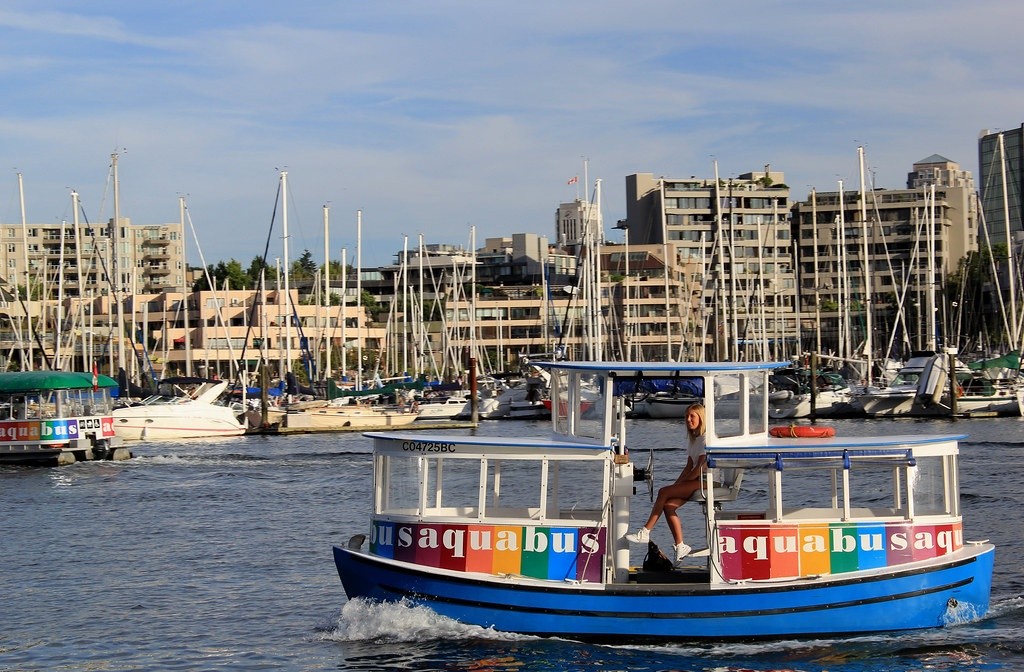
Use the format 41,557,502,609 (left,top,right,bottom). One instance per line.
331,359,997,639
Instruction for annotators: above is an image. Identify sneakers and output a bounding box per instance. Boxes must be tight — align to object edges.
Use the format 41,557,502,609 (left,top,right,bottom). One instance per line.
672,541,692,568
625,526,651,543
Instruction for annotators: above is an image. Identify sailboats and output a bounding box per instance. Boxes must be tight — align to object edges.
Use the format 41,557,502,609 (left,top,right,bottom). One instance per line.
0,132,1024,468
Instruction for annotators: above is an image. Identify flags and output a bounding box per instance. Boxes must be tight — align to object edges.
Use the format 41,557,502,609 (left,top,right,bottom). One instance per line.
92,360,99,391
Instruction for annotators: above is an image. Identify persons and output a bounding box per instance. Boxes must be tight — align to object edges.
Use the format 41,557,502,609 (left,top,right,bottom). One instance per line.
191,387,198,400
70,410,76,417
625,403,726,569
525,370,545,404
397,395,406,414
84,405,95,416
32,412,39,418
408,395,419,414
248,373,258,388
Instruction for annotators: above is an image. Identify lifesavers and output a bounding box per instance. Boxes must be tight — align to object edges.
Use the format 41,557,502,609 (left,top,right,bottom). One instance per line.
769,425,836,439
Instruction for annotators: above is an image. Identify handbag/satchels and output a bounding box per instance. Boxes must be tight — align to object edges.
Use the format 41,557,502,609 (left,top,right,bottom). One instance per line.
643,541,673,570
